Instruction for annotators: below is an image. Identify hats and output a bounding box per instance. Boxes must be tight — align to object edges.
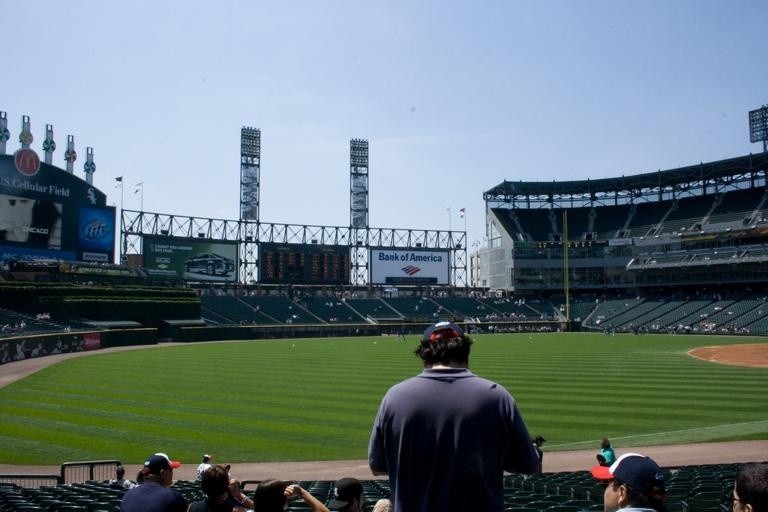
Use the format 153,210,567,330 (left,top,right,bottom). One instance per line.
536,435,546,442
591,453,665,500
144,452,181,473
327,477,363,509
423,321,464,340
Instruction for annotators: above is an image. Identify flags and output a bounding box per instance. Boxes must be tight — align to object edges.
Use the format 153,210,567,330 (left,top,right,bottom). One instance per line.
133,181,142,193
114,176,123,189
459,208,465,217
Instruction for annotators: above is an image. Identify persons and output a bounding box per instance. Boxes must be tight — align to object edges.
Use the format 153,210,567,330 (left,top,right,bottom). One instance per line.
595,438,615,488
731,461,768,512
1,257,767,366
523,436,546,480
590,454,672,512
369,320,541,510
111,447,392,512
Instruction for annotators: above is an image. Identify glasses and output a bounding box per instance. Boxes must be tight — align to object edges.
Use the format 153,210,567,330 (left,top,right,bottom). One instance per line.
728,491,742,502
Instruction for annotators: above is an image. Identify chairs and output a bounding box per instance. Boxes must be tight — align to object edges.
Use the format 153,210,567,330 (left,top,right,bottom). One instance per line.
0,460,768,512
